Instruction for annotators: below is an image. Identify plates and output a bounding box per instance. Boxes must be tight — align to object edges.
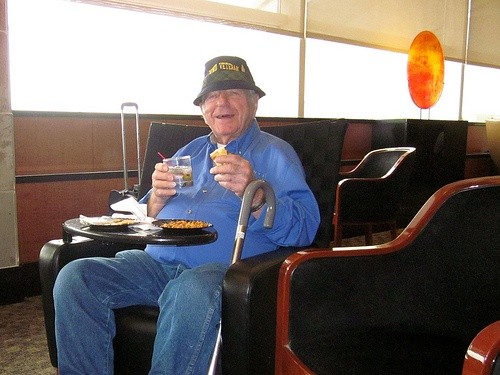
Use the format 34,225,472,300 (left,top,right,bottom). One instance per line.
86,216,142,228
151,218,214,231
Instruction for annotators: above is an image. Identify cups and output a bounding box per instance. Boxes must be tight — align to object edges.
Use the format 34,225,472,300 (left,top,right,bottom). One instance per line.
162,155,194,189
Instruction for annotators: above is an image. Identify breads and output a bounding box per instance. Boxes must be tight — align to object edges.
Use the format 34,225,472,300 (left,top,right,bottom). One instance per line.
210,147,228,165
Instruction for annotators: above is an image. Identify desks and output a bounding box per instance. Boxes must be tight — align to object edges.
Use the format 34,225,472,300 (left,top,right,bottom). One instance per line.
63,217,218,245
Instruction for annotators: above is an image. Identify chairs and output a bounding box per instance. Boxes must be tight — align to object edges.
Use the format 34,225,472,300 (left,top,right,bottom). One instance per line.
39,119,500,375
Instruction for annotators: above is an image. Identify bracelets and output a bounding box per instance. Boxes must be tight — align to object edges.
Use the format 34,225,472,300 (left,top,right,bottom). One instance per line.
250,198,265,213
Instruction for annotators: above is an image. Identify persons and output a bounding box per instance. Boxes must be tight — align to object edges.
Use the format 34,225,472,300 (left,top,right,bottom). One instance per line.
52,56,321,375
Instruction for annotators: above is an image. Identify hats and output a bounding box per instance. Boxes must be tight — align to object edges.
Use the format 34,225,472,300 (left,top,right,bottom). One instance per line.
193,56,266,106
